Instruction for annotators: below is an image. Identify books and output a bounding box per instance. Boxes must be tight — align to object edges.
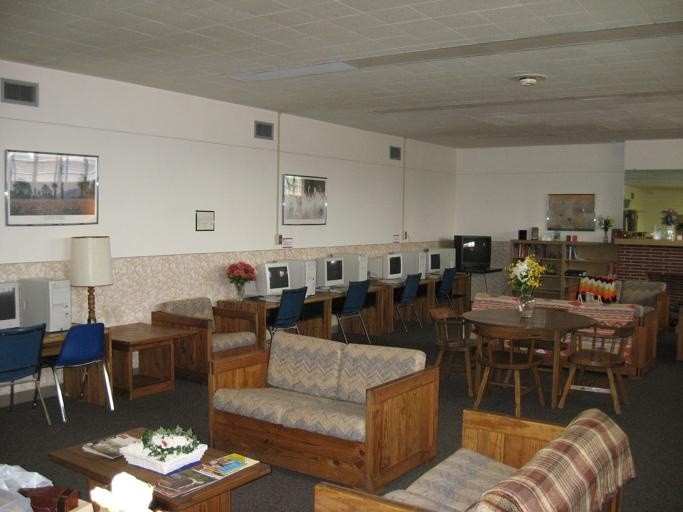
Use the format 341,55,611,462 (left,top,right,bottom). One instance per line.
572,245,577,260
144,467,216,498
577,257,584,261
564,269,586,277
568,246,572,259
193,452,259,480
82,432,141,459
531,227,538,240
512,243,546,259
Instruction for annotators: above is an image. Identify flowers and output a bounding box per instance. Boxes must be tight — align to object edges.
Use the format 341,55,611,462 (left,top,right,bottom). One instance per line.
223,258,259,283
657,207,678,226
592,214,615,232
505,253,548,308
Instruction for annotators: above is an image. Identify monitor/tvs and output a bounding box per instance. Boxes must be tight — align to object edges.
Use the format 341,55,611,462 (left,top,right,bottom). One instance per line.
0,281,20,330
454,234,490,272
426,252,441,279
246,262,289,300
371,254,402,282
314,258,344,291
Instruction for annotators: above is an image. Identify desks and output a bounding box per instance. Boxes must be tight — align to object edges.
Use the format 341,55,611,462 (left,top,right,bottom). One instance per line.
48,424,272,512
1,320,196,406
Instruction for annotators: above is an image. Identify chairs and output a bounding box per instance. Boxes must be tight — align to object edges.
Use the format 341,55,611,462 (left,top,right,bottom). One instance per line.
424,270,676,418
0,321,118,428
274,267,459,346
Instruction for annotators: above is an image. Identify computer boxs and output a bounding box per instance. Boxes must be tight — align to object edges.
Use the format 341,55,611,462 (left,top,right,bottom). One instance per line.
402,252,426,280
435,248,456,275
290,260,316,297
20,277,72,334
333,252,367,293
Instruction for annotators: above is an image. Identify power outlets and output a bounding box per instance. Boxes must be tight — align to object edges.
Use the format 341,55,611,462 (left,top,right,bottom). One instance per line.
276,234,282,244
401,232,407,241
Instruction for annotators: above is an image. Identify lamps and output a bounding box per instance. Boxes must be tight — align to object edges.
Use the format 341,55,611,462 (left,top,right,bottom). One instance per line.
71,236,112,326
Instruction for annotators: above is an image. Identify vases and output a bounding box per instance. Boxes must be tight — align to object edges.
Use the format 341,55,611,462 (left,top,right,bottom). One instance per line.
667,225,673,240
602,232,608,243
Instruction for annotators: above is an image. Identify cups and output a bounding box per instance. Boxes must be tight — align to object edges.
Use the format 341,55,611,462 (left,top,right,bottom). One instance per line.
665,226,676,241
652,223,663,239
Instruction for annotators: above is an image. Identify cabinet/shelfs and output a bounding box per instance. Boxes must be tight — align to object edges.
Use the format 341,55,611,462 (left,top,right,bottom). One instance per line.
510,238,602,300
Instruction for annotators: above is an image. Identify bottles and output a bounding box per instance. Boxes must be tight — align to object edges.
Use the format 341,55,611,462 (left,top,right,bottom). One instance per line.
564,226,571,242
553,223,560,242
623,230,646,239
584,291,594,306
571,230,577,243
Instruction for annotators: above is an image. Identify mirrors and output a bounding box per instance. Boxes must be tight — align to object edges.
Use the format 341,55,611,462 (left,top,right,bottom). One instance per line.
622,170,682,241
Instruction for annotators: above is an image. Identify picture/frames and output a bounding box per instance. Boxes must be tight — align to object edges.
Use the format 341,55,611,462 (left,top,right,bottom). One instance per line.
4,148,98,226
281,173,326,226
193,210,216,233
544,191,594,232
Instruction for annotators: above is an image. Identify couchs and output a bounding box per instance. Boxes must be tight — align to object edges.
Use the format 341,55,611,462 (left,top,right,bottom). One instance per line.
204,330,446,495
312,405,637,511
